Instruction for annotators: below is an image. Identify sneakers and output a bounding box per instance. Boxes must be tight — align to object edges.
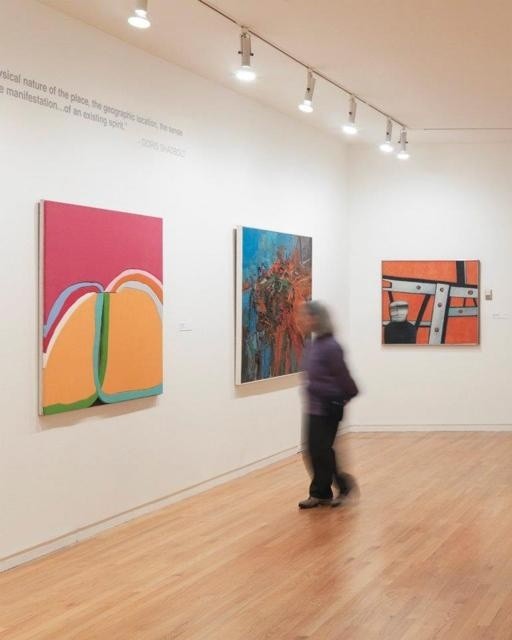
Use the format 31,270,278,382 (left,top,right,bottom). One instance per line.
299,497,333,508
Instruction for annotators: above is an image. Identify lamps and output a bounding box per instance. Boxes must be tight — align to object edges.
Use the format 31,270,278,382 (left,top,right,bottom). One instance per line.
127,0,415,163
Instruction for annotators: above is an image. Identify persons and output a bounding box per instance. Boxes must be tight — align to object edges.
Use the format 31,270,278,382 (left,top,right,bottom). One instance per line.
297,301,358,509
